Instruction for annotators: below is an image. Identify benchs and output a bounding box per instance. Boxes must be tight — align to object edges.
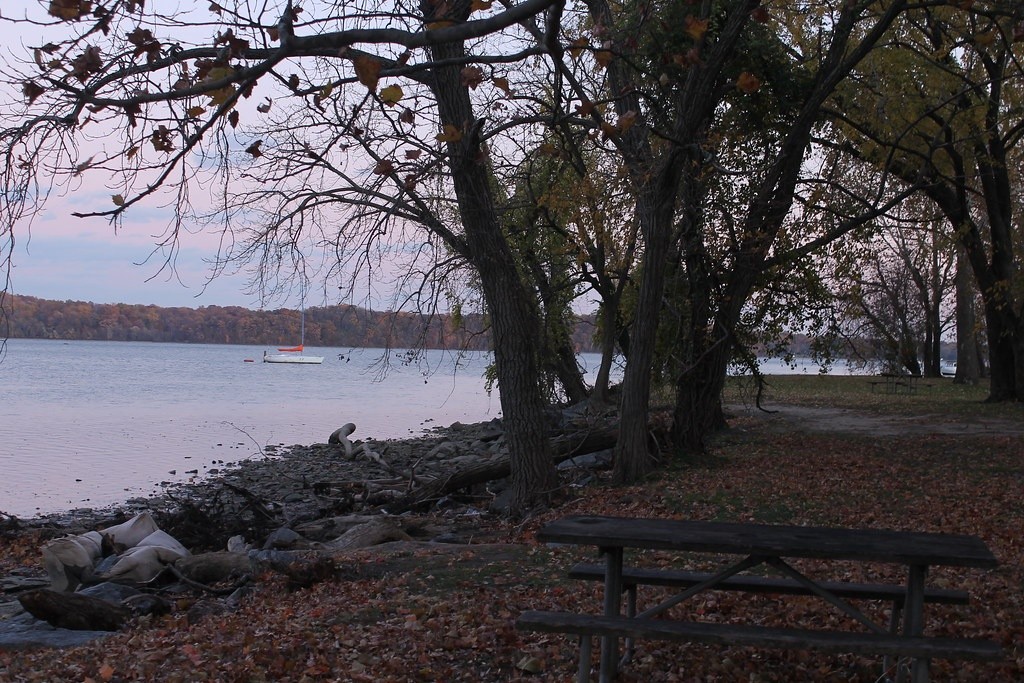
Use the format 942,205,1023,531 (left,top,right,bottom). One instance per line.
867,382,904,393
516,612,1002,683
903,384,936,397
568,561,969,678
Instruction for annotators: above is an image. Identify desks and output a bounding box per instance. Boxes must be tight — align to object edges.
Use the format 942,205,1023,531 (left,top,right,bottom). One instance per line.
881,373,922,395
536,515,998,683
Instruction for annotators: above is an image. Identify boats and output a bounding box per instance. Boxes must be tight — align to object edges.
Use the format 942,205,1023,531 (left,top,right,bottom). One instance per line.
264,350,325,364
942,360,957,375
